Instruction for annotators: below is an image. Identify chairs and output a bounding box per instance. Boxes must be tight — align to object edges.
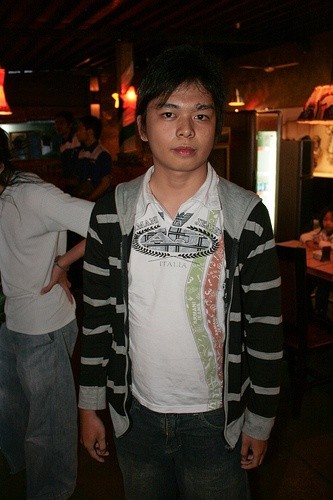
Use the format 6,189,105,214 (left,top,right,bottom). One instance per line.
275,244,333,417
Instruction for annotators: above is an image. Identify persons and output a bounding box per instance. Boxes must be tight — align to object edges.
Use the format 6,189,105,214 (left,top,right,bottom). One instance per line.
298,207,333,322
75,45,283,500
0,126,99,499
13,115,113,292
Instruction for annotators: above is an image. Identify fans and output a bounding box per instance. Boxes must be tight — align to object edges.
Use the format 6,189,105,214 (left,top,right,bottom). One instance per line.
239,47,301,74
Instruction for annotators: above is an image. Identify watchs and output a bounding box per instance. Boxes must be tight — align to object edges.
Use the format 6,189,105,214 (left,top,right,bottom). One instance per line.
53,255,71,272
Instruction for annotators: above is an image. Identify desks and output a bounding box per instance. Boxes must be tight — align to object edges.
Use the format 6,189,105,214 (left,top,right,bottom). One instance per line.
276,240,333,290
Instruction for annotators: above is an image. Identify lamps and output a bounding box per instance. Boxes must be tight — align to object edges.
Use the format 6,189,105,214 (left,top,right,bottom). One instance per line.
0,65,13,116
